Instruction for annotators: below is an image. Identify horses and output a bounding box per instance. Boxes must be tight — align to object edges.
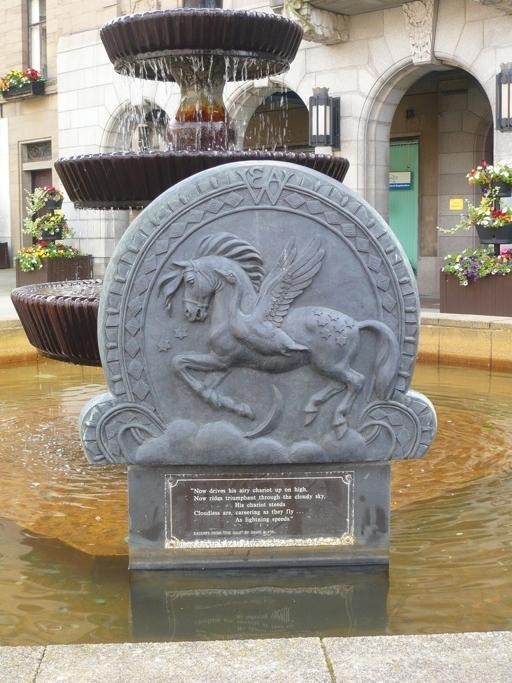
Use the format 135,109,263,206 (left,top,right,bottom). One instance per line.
157,229,400,439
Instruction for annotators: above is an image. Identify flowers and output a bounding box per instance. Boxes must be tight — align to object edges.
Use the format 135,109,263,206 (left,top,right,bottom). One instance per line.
15,184,80,272
435,161,512,287
0,67,46,92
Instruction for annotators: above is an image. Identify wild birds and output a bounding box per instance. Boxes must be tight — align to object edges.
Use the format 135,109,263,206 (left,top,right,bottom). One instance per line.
213,235,325,357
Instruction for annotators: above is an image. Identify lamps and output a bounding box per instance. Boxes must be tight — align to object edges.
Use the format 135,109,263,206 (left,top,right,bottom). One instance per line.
496,62,512,132
309,86,341,152
